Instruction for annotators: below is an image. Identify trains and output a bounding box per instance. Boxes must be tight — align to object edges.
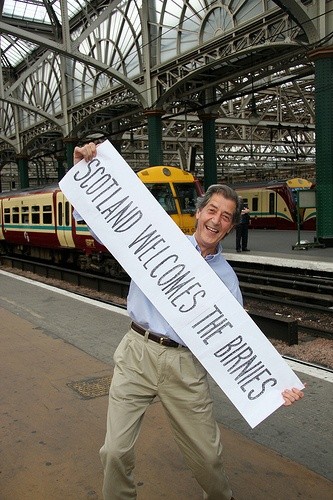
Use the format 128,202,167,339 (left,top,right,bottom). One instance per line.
0,164,207,281
214,176,318,230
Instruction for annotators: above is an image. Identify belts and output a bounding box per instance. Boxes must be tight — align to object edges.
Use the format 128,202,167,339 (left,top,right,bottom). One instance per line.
131,321,185,348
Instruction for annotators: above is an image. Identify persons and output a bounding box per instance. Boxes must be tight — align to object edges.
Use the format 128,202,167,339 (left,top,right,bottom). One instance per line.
234,195,254,254
73,141,308,500
